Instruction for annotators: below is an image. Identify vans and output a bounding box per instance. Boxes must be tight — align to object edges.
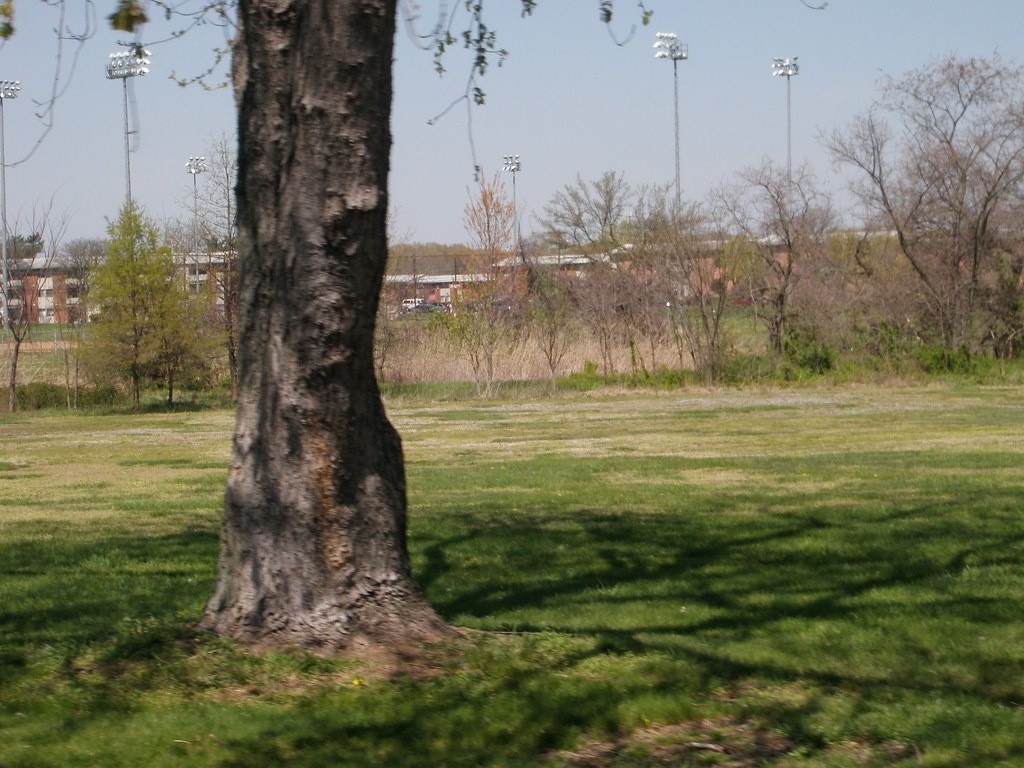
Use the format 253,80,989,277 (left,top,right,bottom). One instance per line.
401,298,424,312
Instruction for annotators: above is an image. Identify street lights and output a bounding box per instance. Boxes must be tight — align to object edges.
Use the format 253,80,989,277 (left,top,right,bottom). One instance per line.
184,156,207,294
653,31,689,248
104,47,153,315
1,78,22,336
771,54,801,242
501,153,522,270
